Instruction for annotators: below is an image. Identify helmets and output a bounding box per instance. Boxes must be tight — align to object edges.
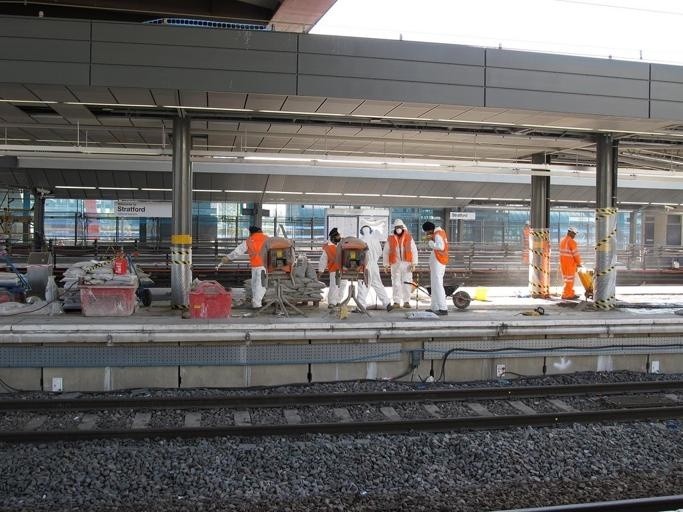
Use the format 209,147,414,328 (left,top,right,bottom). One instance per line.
568,227,577,234
393,219,405,228
525,220,530,224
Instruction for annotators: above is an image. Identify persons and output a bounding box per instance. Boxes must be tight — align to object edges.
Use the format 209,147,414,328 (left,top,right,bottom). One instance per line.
350,227,394,313
316,227,343,310
421,222,449,315
521,220,531,263
560,226,582,300
258,227,264,234
383,219,419,308
221,226,270,310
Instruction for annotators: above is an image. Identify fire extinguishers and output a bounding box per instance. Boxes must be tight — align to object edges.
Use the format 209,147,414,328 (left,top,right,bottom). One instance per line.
113,246,126,275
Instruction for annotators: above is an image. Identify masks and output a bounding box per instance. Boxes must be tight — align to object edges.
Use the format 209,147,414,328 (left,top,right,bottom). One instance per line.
396,228,402,234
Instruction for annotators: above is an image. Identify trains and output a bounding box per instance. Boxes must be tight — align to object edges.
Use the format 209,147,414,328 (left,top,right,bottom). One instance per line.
43,199,217,256
286,207,642,248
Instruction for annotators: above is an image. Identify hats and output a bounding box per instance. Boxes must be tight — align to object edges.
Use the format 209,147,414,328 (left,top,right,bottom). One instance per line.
329,228,337,235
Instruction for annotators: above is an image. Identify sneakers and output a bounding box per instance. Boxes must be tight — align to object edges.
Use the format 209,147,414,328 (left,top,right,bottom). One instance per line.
387,302,411,308
329,304,334,309
253,304,262,308
352,309,361,313
425,309,449,315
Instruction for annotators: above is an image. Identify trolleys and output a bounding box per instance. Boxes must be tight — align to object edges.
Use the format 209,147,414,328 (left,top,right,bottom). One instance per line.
404,272,471,309
